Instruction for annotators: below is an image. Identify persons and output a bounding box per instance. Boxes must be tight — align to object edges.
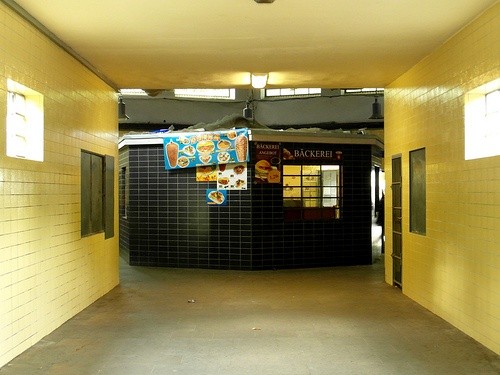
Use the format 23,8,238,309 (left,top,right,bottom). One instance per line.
375,189,385,254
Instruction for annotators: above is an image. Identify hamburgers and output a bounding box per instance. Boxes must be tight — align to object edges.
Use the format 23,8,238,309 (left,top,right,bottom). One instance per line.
197,141,214,152
255,160,272,173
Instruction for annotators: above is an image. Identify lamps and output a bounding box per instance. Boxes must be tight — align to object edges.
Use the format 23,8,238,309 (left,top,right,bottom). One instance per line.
243,102,252,118
118,96,126,118
368,87,381,119
